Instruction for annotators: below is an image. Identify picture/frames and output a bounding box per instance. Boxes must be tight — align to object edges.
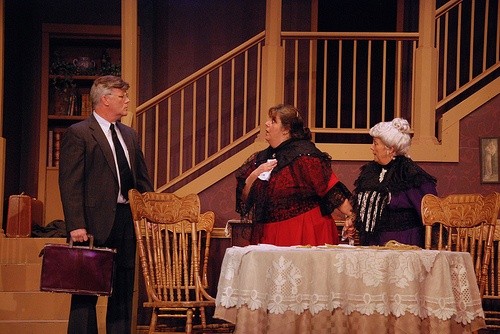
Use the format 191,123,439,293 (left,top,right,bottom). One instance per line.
479,136,500,186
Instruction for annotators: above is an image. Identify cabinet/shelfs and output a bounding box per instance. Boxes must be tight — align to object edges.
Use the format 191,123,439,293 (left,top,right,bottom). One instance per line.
19,24,142,231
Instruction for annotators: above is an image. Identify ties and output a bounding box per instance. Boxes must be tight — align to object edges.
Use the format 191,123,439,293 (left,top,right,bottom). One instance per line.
109,124,134,202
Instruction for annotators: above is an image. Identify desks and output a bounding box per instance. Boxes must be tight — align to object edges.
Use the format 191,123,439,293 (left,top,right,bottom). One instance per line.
213,244,486,334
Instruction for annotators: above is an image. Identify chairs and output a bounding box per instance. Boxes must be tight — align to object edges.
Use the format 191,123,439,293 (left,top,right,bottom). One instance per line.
421,191,500,334
128,190,235,334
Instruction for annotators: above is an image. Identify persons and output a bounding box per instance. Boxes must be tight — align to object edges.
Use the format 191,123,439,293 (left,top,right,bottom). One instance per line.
59,76,155,334
236,104,453,248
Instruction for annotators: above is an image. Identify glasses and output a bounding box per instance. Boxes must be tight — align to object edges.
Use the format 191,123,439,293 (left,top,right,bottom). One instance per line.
105,91,129,100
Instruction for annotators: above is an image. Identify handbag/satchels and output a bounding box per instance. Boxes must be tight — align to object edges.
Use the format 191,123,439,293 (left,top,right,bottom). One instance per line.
39,234,116,296
